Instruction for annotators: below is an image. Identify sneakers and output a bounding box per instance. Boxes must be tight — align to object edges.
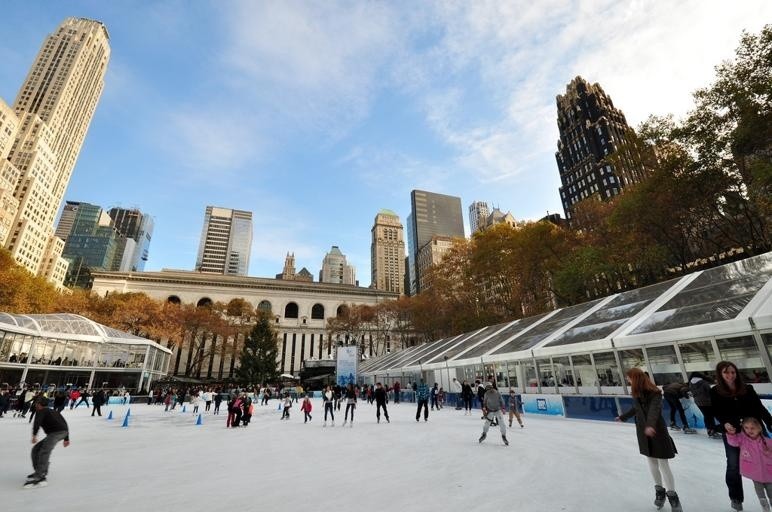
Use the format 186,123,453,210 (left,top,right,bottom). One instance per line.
23,472,48,485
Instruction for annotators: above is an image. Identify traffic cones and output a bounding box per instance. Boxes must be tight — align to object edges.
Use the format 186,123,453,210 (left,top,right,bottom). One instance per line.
182,406,186,412
195,413,203,426
121,416,128,427
277,402,283,410
107,410,113,421
126,407,131,417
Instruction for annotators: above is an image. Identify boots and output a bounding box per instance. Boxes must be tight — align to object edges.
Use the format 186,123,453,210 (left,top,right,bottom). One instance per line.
653,485,667,506
479,432,488,441
501,434,509,444
667,490,683,512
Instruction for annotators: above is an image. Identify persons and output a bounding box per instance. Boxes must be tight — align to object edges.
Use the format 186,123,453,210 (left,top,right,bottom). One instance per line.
726,417,772,512
322,383,360,424
615,367,683,512
479,385,508,445
453,376,496,419
148,386,187,410
408,379,443,421
189,384,312,427
23,396,70,489
542,375,582,387
362,381,401,422
703,361,772,511
598,374,614,385
509,390,524,428
744,370,769,382
1,383,147,422
662,372,723,439
0,350,144,369
